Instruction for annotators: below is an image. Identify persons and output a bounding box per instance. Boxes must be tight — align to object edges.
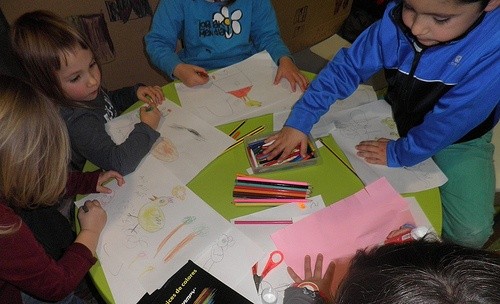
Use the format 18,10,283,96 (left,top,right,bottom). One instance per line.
0,73,126,304
263,0,500,253
283,222,500,304
5,10,166,223
143,0,312,95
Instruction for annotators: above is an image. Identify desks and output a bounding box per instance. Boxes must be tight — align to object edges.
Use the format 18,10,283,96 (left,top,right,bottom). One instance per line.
75,70,442,304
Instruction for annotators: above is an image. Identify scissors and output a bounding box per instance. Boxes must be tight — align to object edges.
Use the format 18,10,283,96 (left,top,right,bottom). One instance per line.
252,251,284,293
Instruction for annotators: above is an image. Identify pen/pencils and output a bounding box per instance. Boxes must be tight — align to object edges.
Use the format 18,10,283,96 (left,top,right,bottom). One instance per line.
234,221,293,224
84,206,88,212
233,173,313,206
147,107,152,111
